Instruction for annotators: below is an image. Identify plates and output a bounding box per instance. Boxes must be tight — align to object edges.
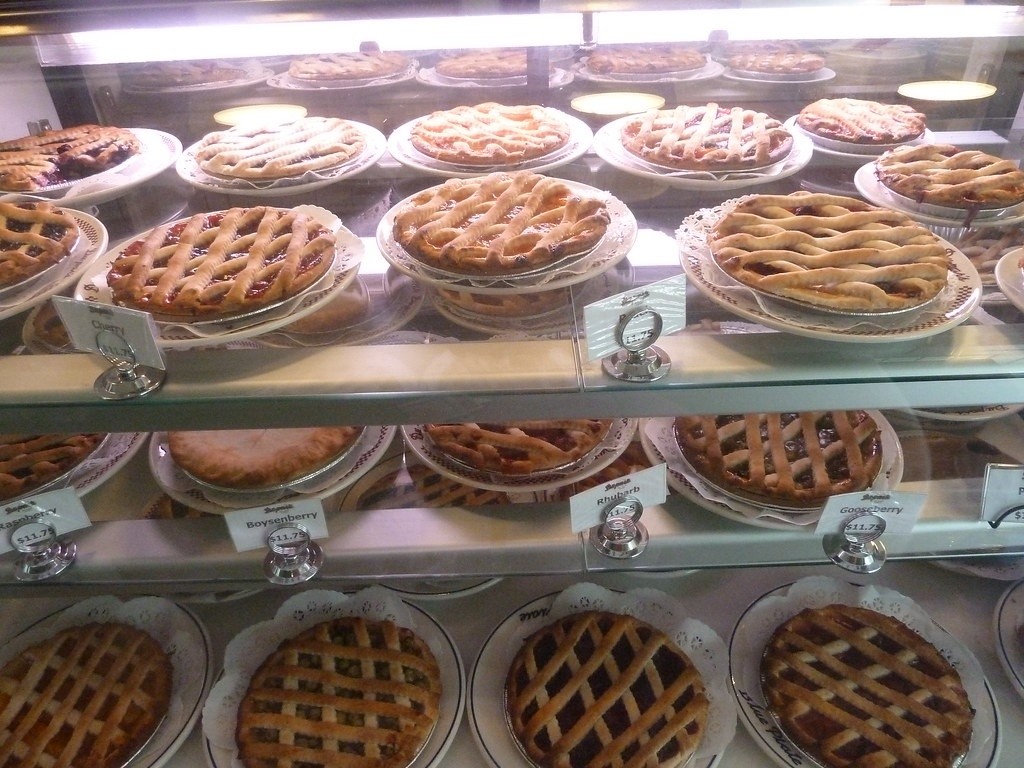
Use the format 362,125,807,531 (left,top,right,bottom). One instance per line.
593,108,816,193
724,579,1000,768
200,587,466,768
336,451,512,603
992,576,1024,703
374,173,642,295
781,111,936,159
993,246,1024,314
885,396,1022,428
38,430,152,507
468,590,730,768
879,428,1024,580
639,409,904,531
140,484,285,606
147,422,398,521
121,33,977,99
0,205,107,323
2,590,215,768
74,203,361,350
680,212,984,344
852,158,1024,229
395,413,637,491
245,239,424,350
174,117,388,197
431,256,637,337
385,102,592,181
0,127,183,211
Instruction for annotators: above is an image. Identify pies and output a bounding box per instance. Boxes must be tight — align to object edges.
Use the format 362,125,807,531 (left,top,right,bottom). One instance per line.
121,42,826,89
0,100,1024,764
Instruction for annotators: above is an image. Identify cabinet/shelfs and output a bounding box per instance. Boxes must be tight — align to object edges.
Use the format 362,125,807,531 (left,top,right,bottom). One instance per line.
0,0,1024,768
